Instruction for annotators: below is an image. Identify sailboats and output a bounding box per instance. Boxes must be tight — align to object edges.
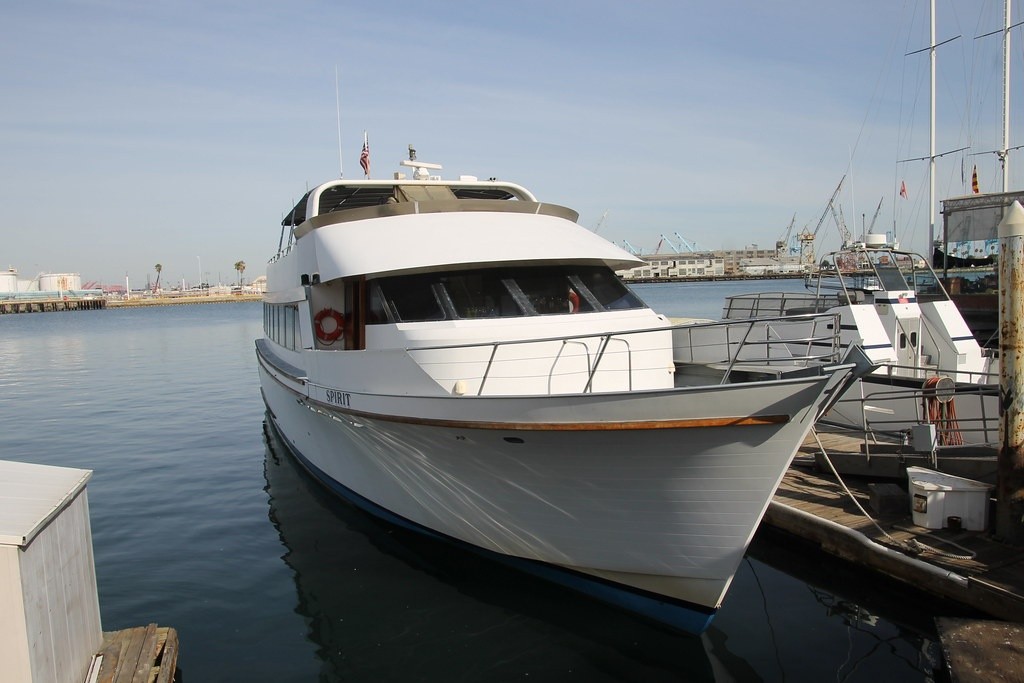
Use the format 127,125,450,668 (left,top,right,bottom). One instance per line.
663,0,1023,443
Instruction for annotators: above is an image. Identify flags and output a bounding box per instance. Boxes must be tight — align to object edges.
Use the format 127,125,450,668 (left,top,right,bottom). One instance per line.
900,181,907,199
972,165,979,193
360,133,371,174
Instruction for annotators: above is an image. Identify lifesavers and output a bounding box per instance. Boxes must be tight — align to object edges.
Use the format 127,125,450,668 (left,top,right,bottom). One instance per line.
568,292,580,314
314,309,345,341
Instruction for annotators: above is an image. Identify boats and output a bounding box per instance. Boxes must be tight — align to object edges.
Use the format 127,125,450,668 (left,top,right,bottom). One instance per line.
253,60,892,641
